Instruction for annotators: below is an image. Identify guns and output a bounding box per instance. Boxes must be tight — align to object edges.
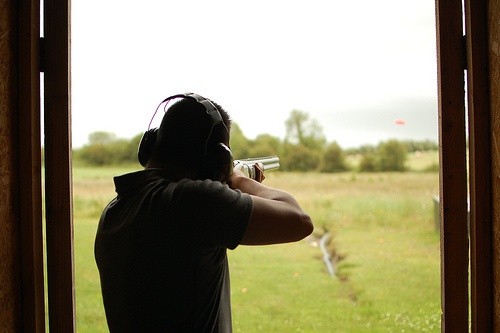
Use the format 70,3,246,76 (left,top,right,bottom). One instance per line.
232,156,280,178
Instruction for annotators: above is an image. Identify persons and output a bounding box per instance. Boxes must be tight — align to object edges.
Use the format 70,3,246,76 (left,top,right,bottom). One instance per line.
94,89,314,332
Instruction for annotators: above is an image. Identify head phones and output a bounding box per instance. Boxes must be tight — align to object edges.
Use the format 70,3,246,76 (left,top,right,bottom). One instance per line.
137,94,233,182
